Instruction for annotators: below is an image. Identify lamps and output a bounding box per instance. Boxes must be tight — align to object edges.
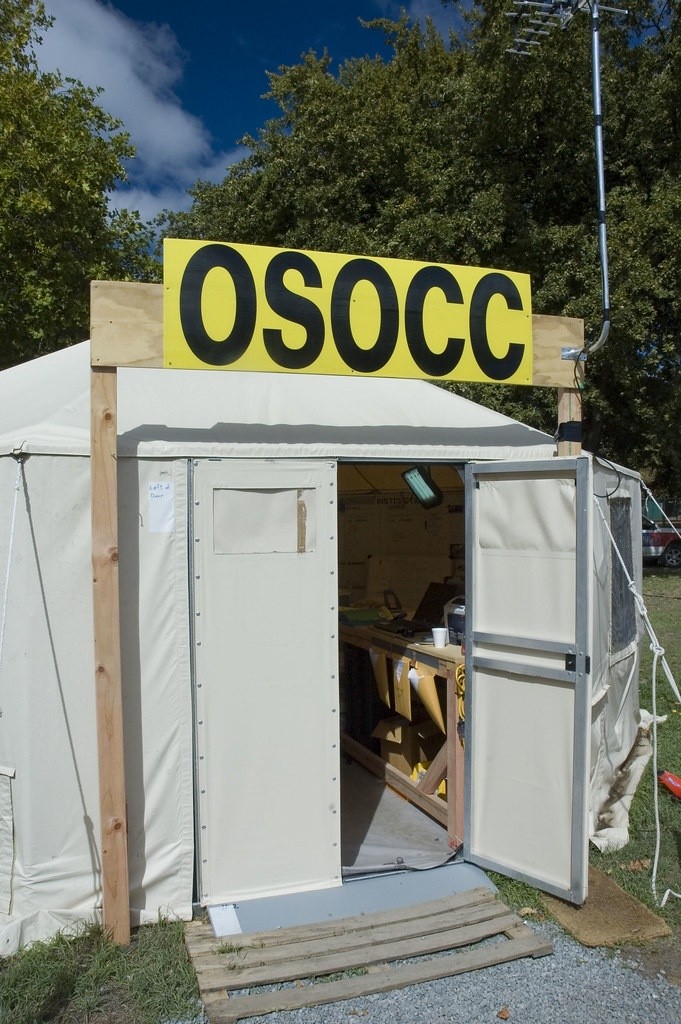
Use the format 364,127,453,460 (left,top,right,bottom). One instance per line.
400,466,444,510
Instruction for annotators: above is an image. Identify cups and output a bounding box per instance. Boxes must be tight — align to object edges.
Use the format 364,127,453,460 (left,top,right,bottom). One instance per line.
431,628,447,647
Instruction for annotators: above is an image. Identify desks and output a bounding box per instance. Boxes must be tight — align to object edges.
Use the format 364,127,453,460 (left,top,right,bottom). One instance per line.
339,620,465,851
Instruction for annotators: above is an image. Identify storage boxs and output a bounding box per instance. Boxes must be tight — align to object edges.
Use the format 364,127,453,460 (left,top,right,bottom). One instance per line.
371,710,446,775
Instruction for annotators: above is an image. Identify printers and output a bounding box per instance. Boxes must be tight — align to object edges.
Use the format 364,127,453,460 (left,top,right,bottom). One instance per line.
444,595,465,647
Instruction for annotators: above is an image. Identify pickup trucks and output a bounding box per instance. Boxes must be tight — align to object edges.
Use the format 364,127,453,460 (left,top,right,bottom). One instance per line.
641,514,681,569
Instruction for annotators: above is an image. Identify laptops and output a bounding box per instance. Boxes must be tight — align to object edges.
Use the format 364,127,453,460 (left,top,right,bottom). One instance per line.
373,581,459,634
